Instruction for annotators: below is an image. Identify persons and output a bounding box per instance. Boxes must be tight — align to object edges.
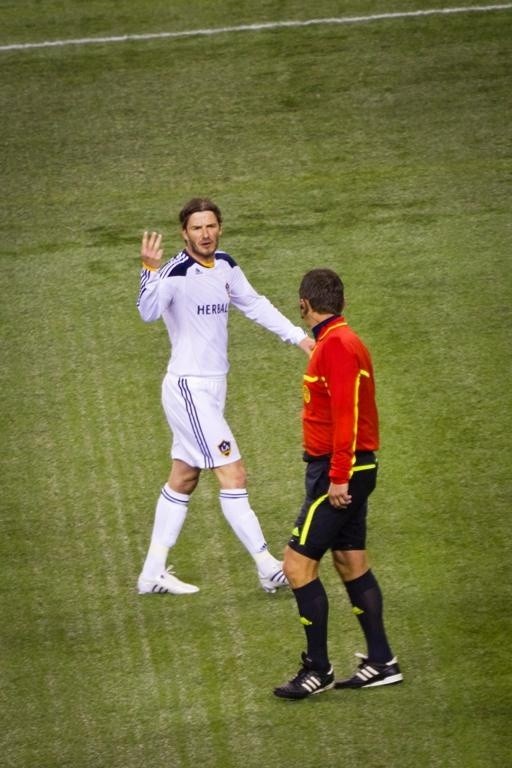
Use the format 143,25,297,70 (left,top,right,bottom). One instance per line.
137,196,317,596
263,269,403,700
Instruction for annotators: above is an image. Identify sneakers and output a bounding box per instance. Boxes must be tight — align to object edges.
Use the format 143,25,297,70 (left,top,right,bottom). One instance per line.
136,570,201,596
256,559,291,594
333,651,405,692
271,652,337,700
